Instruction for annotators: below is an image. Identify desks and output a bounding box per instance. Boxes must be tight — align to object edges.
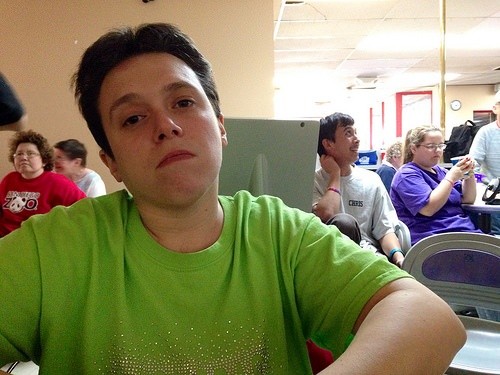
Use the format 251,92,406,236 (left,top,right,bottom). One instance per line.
461,200,500,234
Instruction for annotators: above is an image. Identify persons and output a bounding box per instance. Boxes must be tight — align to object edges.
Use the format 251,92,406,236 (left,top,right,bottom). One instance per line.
0,129,88,238
312,111,405,269
389,124,483,247
376,137,403,193
0,72,29,131
53,139,106,198
468,90,500,236
0,22,467,375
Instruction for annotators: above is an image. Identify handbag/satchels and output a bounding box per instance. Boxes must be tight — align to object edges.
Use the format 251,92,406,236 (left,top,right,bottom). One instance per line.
445,120,476,154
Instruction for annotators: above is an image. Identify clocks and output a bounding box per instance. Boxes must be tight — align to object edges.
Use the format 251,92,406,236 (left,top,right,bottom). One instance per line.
450,100,461,111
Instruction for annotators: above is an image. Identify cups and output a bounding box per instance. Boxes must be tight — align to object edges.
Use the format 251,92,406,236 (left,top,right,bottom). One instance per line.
451,157,469,175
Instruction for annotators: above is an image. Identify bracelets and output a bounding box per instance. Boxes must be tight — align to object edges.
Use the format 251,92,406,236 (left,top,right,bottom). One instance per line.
444,178,454,187
388,248,405,258
327,187,340,194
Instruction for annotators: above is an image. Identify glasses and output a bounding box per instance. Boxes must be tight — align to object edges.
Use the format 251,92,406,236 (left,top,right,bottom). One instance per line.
13,151,41,158
417,143,447,151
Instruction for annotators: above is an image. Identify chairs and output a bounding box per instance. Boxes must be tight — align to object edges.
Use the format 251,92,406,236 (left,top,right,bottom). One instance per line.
397,221,500,375
